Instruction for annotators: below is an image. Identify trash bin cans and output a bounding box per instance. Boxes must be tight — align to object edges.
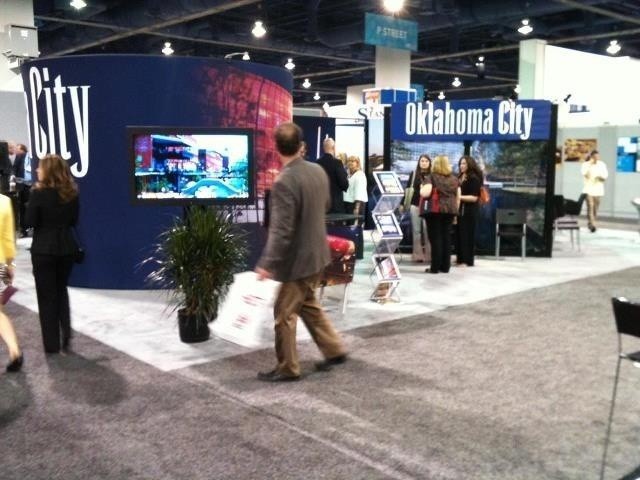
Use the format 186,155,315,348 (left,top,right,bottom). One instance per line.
321,214,364,260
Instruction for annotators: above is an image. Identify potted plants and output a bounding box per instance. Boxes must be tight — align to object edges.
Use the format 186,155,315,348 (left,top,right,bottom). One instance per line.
131,196,253,342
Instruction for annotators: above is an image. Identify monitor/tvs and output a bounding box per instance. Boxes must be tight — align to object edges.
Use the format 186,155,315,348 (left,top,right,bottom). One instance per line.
126,125,255,207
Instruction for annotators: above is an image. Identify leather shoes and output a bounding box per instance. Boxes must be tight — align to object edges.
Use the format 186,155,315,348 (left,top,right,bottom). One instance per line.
315,356,348,371
7,356,23,372
257,370,301,381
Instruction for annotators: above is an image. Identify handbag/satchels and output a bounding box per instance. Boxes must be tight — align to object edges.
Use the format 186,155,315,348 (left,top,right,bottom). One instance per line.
418,187,440,217
403,186,414,211
75,240,85,263
0,286,18,305
478,186,490,205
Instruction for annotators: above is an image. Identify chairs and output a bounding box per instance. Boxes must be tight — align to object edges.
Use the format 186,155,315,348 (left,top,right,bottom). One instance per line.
599,298,639,479
495,208,527,258
564,193,586,215
553,194,580,253
316,212,365,315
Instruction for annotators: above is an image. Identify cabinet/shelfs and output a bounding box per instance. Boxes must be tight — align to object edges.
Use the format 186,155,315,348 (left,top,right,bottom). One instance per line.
369,170,404,302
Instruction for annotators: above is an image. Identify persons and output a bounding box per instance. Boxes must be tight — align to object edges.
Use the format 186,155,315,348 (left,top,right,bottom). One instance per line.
419,155,462,273
297,134,367,215
397,156,434,265
26,154,82,352
577,149,609,234
0,194,24,372
255,124,348,382
1,141,39,239
455,156,483,266
